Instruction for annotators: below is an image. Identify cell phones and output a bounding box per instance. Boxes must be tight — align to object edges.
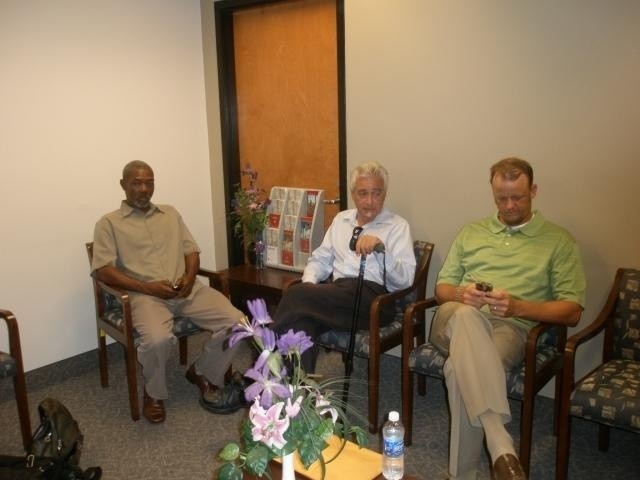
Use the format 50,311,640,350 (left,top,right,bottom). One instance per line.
476,281,493,292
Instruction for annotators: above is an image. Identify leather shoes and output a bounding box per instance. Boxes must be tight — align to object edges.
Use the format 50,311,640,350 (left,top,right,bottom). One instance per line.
489,453,527,480
185,362,216,390
142,385,166,424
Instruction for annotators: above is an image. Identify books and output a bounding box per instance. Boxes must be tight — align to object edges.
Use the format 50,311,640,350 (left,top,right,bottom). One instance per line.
271,433,388,480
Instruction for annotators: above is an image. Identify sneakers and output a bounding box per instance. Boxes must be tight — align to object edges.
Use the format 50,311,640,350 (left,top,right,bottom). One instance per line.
200,371,254,414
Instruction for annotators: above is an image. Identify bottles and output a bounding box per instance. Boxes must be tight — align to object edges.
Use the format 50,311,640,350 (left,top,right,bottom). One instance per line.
382,412,404,480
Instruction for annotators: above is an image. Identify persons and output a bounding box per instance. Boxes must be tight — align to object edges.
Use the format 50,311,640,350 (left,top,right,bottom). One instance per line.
430,156,587,480
201,164,418,415
90,162,248,424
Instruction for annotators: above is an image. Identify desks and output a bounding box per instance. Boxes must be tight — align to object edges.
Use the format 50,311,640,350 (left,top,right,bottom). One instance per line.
214,426,420,480
216,264,304,385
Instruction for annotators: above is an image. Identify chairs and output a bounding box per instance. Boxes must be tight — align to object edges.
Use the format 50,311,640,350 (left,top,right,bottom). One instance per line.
281,239,436,435
0,308,40,457
85,241,227,421
555,268,639,479
400,294,572,480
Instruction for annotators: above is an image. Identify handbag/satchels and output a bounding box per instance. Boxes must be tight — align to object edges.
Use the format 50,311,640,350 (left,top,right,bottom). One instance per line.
0,455,71,479
29,397,84,465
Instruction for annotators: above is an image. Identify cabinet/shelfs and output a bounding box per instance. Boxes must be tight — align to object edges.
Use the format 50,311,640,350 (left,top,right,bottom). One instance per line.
262,186,325,272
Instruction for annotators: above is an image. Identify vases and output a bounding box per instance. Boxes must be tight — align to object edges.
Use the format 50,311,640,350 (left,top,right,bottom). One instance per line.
253,240,264,266
281,449,297,480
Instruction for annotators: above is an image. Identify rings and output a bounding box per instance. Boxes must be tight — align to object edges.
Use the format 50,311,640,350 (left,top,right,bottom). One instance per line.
494,305,498,311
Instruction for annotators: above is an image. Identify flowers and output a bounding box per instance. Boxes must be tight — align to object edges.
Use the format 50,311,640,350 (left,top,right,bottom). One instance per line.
210,296,374,480
228,164,272,269
